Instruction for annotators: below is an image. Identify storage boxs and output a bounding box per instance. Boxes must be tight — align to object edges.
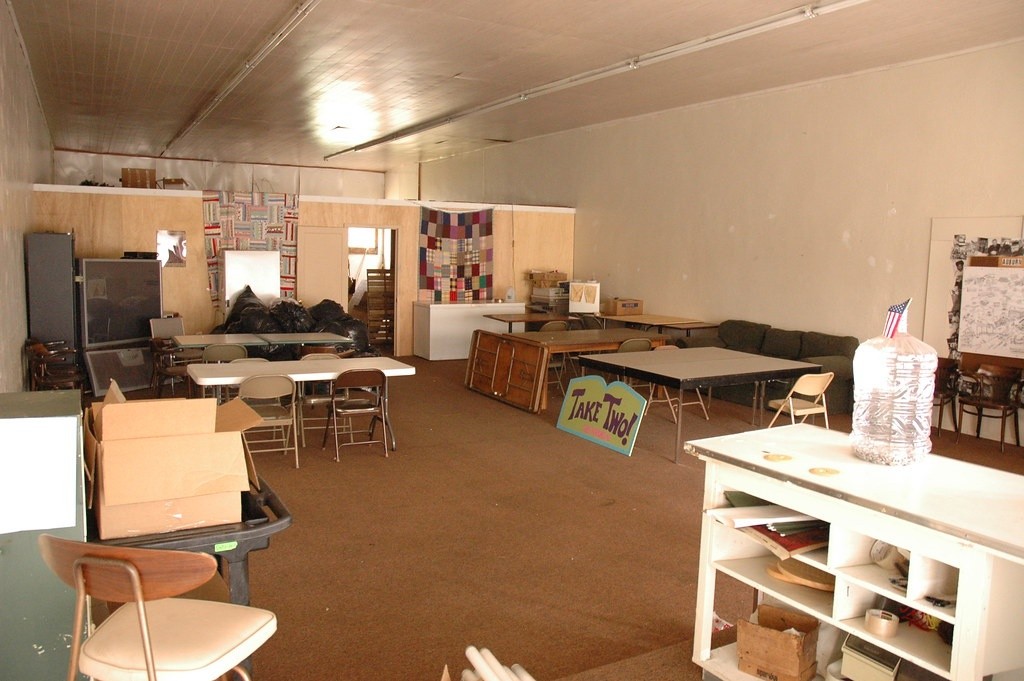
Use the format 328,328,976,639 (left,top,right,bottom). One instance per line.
737,605,822,677
528,273,567,281
83,378,265,541
156,177,189,189
605,297,643,316
122,168,156,189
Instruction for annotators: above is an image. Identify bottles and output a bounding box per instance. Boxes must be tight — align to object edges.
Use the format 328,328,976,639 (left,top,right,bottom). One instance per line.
849,310,937,466
505,285,516,303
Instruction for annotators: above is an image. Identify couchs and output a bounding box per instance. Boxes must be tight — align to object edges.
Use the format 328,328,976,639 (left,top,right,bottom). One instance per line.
675,319,860,419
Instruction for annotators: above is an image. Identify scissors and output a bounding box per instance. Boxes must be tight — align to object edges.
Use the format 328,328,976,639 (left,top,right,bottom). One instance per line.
926,596,956,607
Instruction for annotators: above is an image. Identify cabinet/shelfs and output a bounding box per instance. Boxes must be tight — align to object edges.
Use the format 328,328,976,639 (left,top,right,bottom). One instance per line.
682,423,1024,681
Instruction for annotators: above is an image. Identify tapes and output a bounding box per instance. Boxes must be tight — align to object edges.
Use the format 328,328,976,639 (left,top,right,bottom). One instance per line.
869,540,904,569
864,609,900,636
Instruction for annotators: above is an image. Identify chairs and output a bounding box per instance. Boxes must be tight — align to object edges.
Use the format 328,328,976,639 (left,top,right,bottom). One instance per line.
296,353,354,448
38,534,279,681
607,338,653,393
321,368,389,462
201,343,247,400
539,321,567,398
149,317,209,396
581,314,615,382
932,357,961,438
644,346,710,424
767,371,834,429
25,336,81,393
148,337,206,399
238,373,300,470
953,363,1024,454
222,357,286,451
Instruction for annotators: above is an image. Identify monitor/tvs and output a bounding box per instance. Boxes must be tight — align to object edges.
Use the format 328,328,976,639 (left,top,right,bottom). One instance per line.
77,258,163,350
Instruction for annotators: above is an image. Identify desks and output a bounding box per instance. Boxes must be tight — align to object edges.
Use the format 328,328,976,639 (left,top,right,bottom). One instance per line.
186,357,416,452
598,313,720,338
624,355,823,464
413,299,527,360
483,314,580,334
256,332,354,409
503,328,672,401
171,333,269,400
578,347,760,426
463,329,550,415
